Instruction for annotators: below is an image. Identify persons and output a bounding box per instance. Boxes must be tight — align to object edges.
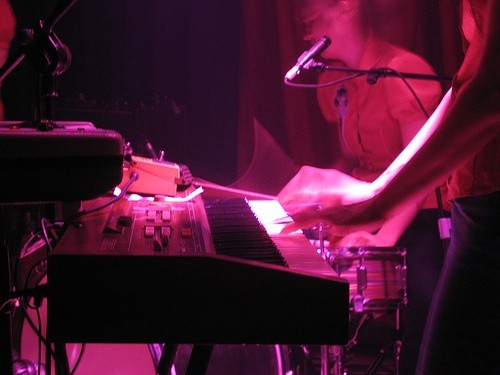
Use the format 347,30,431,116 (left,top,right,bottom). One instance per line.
297,0,459,268
276,0,500,374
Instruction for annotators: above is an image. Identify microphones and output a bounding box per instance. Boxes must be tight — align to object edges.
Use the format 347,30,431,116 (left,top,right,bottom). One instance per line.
17,28,72,74
285,36,330,82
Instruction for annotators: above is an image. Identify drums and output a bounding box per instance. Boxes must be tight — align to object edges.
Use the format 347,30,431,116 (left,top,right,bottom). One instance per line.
317,241,408,312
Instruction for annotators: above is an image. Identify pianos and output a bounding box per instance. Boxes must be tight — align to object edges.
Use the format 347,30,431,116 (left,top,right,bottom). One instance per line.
48,156,349,344
